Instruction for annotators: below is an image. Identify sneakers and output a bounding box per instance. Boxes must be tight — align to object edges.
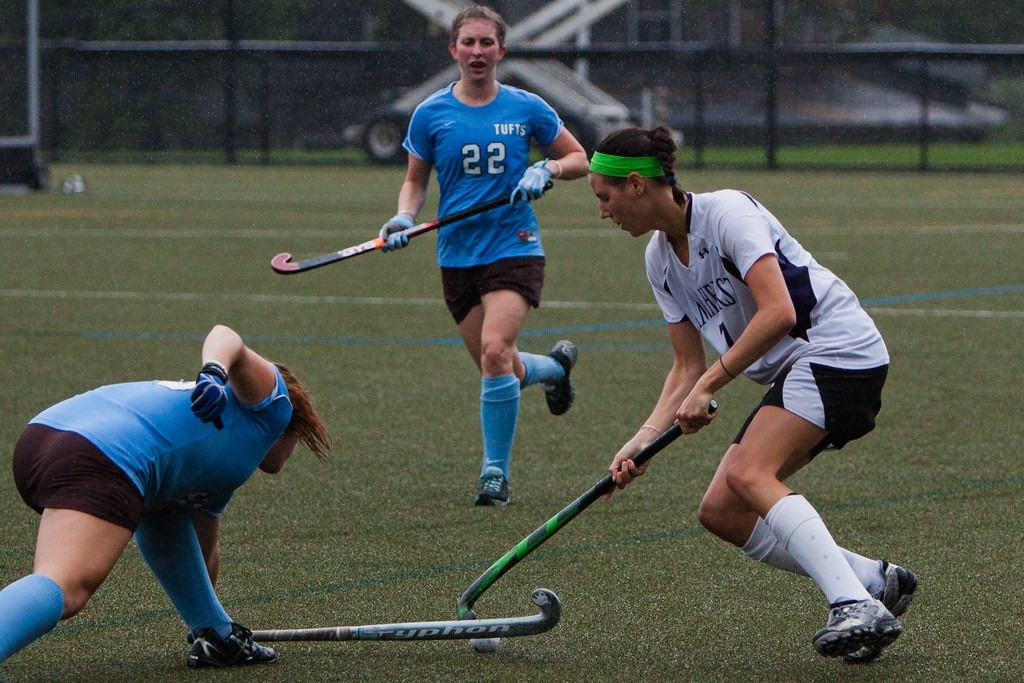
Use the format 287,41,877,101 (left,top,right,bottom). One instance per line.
811,597,902,657
844,559,917,665
474,472,511,507
538,339,577,417
186,620,275,669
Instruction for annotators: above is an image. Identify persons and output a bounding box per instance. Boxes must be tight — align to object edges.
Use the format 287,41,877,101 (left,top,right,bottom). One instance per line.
587,126,918,658
378,5,591,507
0,325,327,667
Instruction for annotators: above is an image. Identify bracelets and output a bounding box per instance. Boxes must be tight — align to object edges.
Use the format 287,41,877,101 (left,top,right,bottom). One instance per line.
640,425,661,436
552,160,562,177
203,362,228,381
719,357,736,379
398,210,415,218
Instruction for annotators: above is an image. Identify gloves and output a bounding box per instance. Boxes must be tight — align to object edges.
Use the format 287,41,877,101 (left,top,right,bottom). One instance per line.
190,362,228,429
509,158,554,208
379,215,413,253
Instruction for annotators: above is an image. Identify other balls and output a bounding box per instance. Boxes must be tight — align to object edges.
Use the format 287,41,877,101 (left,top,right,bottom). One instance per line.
469,637,501,653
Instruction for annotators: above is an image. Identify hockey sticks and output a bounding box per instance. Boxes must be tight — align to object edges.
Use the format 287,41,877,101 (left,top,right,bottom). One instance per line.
186,588,563,645
269,180,555,276
455,398,718,621
